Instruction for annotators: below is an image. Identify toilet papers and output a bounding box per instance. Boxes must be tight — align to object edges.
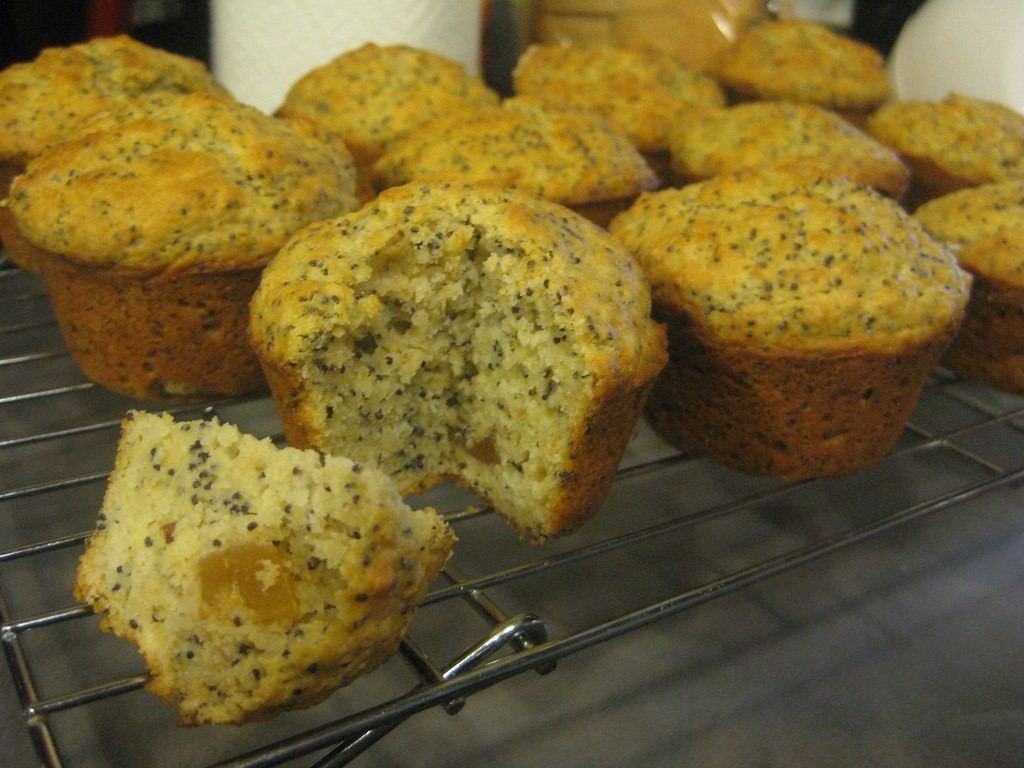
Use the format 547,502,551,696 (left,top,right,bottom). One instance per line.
210,0,484,118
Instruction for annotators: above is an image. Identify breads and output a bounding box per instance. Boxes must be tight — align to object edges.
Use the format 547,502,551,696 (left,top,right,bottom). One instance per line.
0,25,1024,726
